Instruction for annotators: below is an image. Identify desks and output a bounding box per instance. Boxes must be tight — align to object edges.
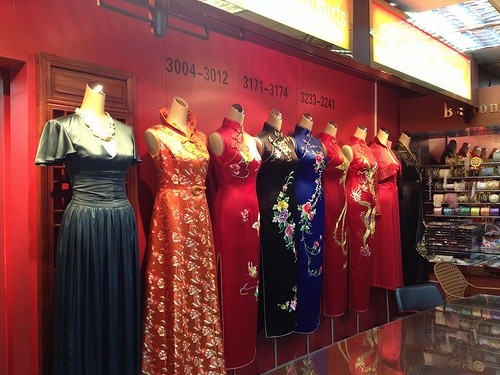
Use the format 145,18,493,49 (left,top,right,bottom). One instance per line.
262,303,500,375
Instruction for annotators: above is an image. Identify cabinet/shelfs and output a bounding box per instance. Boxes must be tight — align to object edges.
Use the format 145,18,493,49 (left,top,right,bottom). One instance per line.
420,161,500,254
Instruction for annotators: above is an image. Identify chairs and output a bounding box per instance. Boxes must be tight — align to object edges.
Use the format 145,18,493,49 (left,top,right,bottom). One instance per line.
433,261,500,304
395,284,445,316
